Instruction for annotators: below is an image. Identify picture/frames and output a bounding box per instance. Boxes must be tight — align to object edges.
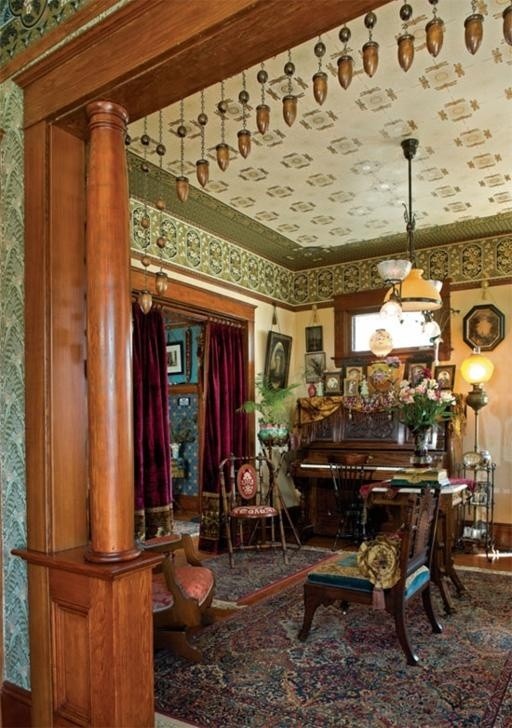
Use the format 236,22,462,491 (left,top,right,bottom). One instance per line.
304,325,455,396
262,331,294,395
165,341,183,376
462,304,505,351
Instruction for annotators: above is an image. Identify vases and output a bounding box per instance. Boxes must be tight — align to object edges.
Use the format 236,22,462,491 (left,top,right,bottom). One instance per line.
168,442,188,479
409,426,433,468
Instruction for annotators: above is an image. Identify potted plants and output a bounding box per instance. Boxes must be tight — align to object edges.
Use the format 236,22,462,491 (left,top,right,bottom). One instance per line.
234,372,303,446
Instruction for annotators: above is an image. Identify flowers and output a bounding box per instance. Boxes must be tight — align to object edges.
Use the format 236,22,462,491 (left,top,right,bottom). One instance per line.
379,367,458,434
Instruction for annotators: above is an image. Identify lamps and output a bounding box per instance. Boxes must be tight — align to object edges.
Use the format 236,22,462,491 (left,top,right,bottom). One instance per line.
377,138,443,312
460,347,494,469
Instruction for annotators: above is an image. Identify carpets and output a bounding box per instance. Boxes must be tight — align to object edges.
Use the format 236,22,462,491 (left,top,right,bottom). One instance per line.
152,551,512,721
188,543,335,600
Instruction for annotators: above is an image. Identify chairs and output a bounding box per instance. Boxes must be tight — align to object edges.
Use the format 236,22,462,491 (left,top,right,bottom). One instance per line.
296,487,443,666
217,458,290,567
327,461,367,551
383,494,471,616
139,533,215,664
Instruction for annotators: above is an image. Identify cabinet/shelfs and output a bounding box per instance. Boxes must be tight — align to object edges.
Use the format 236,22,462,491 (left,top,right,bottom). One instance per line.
454,461,499,563
290,393,467,569
